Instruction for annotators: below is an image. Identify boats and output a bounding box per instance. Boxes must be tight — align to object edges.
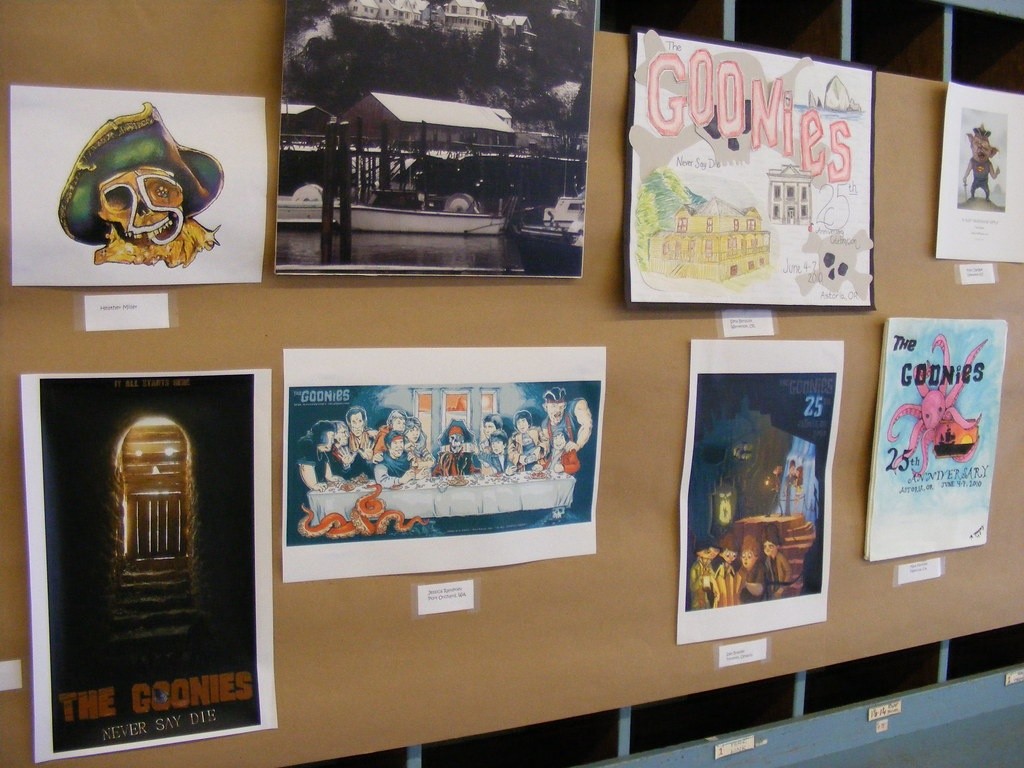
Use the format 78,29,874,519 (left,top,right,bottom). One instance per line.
277,93,585,259
